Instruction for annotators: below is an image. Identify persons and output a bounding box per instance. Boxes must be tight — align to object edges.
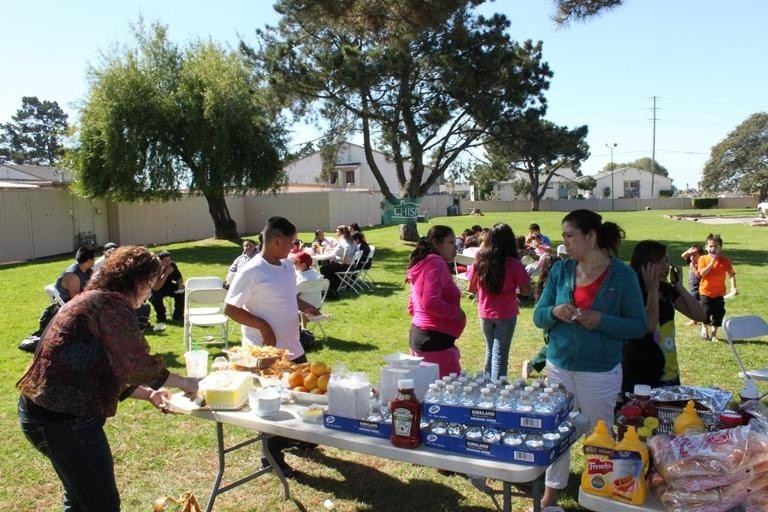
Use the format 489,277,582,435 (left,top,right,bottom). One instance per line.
533,235,552,267
94,242,167,333
223,216,321,475
528,223,551,246
681,244,704,325
525,208,649,512
454,223,489,254
312,229,331,254
149,250,186,322
55,244,96,303
467,222,531,378
698,234,738,343
223,240,262,290
320,225,358,299
521,255,561,378
406,224,466,380
352,233,372,270
348,222,361,233
292,251,322,314
629,240,707,388
15,244,199,512
515,236,540,267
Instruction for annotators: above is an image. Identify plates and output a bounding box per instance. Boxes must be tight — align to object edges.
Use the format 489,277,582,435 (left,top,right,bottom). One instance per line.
222,350,294,369
204,387,256,411
384,352,424,365
162,391,202,414
287,388,329,405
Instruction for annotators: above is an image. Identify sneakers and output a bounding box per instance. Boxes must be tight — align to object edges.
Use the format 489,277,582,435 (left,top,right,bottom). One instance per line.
522,360,530,379
148,323,164,334
261,452,296,477
529,506,552,512
296,441,318,450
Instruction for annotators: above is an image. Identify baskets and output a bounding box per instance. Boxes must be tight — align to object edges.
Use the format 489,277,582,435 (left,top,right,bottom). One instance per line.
652,385,732,435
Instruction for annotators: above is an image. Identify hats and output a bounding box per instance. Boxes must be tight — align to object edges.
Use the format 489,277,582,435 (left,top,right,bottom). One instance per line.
293,253,312,266
103,242,116,249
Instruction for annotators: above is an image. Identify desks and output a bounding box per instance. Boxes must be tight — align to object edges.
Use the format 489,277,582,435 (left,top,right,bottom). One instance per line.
171,370,593,512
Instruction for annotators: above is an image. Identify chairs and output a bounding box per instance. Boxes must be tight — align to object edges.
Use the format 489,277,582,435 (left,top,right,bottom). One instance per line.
336,250,364,294
359,245,376,288
454,254,477,304
184,289,230,351
44,282,64,306
185,277,223,347
723,315,768,403
295,279,330,342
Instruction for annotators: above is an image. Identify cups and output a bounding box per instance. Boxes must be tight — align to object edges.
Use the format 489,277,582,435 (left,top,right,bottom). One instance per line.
184,351,208,379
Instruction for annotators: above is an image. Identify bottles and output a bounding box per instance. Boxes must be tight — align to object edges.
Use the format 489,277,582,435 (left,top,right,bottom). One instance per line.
674,400,703,433
390,379,421,448
614,406,645,441
628,385,657,424
716,412,742,430
425,368,565,415
